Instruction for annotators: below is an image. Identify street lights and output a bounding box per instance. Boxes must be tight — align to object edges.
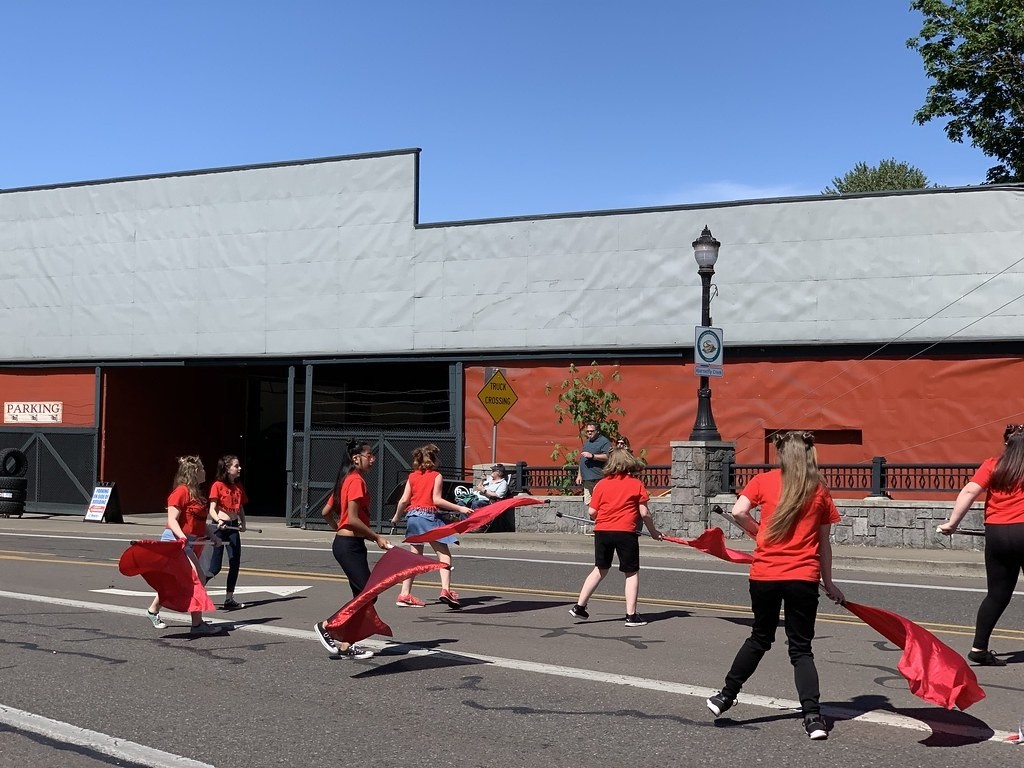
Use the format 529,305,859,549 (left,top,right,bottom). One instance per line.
687,223,722,440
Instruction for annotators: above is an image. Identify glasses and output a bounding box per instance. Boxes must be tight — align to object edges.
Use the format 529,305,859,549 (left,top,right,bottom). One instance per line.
490,470,499,473
617,442,624,445
586,430,596,433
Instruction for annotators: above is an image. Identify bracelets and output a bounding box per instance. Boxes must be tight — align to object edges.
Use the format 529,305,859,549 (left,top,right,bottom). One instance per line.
483,490,487,494
216,518,223,522
591,453,595,460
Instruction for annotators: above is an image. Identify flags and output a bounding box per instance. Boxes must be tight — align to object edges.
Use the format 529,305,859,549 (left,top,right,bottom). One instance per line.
400,497,546,545
839,600,987,713
191,523,220,579
118,539,217,614
664,527,755,564
324,544,450,647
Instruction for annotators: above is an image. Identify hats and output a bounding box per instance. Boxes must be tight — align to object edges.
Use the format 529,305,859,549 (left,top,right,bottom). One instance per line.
490,464,505,473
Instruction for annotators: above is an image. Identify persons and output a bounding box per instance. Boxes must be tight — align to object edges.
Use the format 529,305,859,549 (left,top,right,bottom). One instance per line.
203,454,248,610
706,430,846,741
615,435,634,455
146,455,223,636
936,422,1024,667
390,444,475,608
575,421,613,537
456,463,508,533
568,447,665,627
313,437,392,661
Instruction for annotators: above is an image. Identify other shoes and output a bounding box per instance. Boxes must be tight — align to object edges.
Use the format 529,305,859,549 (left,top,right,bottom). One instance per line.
472,528,484,533
591,531,595,537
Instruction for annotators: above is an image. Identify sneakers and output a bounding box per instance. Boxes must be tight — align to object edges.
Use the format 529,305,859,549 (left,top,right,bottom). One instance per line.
968,649,1006,666
625,612,648,627
568,603,589,619
802,714,828,739
396,594,425,608
706,691,738,717
439,589,461,609
191,621,222,634
145,609,168,629
338,645,373,660
224,597,246,610
314,621,339,655
